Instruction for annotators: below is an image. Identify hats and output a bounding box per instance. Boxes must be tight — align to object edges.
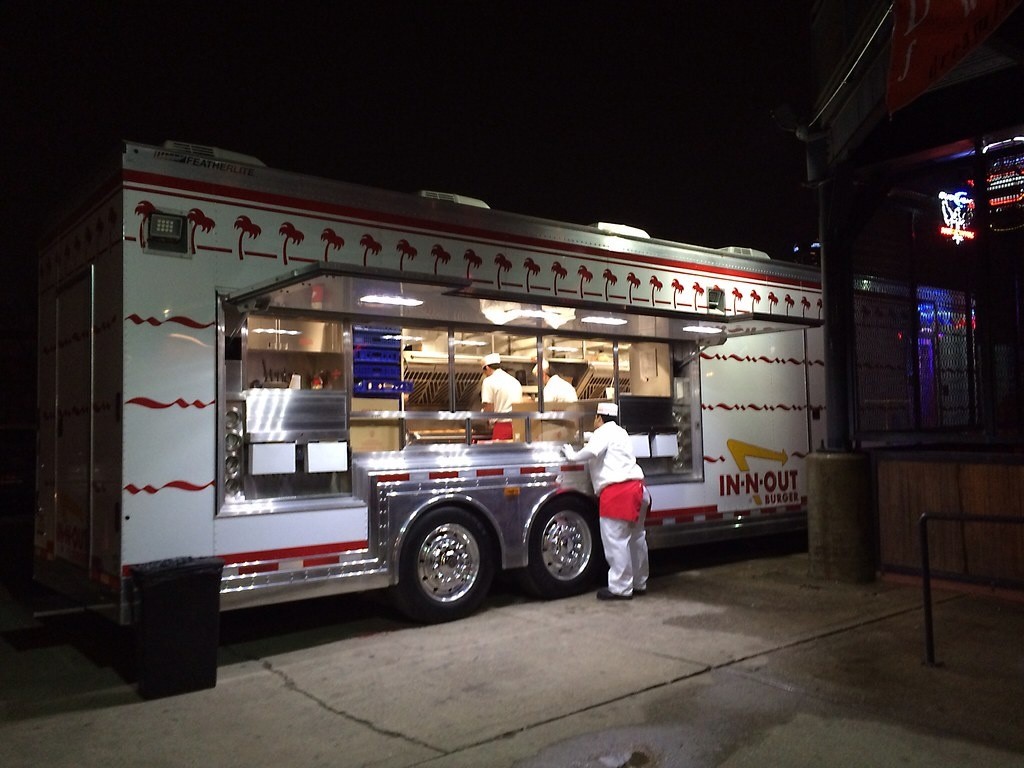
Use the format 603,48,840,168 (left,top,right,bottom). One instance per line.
597,403,618,417
532,358,549,374
482,353,500,368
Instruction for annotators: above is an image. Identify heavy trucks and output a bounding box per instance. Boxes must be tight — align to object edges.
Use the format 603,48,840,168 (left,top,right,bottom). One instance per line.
14,138,1024,628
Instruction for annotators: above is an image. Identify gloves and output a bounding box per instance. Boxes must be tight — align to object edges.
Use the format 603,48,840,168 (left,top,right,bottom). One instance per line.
561,444,574,460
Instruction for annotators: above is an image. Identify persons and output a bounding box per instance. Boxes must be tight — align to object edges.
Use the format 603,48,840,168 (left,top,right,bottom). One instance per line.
561,403,652,600
472,353,522,440
533,361,578,441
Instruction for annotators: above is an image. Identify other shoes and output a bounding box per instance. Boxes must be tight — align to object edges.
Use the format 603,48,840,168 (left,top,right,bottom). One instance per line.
597,588,632,601
632,589,647,595
